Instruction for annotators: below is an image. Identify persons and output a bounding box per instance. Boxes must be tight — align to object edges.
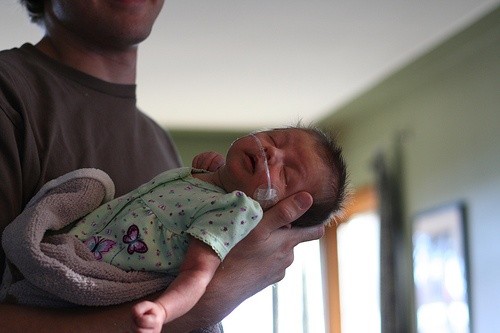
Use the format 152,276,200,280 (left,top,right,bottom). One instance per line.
0,0,325,333
54,117,353,333
